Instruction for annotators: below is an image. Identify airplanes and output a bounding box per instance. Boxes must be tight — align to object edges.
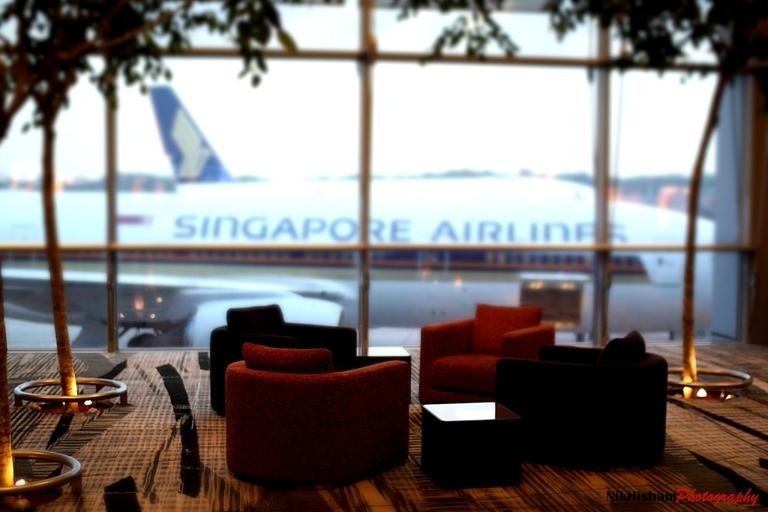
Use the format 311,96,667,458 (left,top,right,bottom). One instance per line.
0,86,717,347
